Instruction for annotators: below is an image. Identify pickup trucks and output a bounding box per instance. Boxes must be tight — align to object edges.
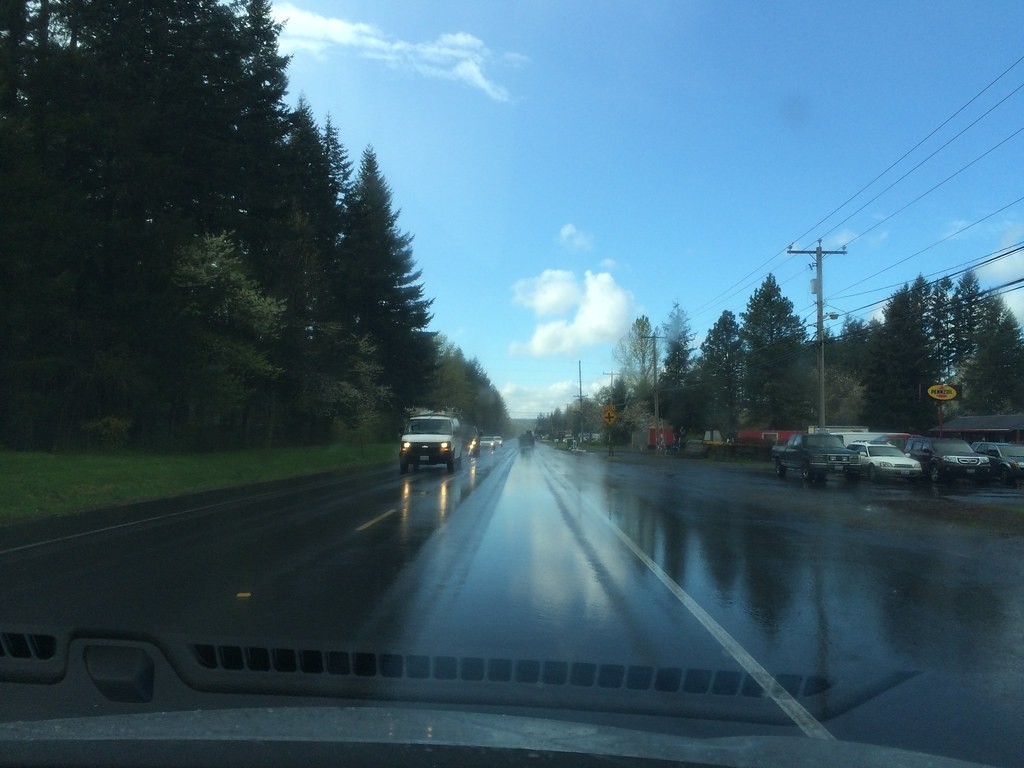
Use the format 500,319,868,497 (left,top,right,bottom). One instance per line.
771,433,862,483
870,433,927,451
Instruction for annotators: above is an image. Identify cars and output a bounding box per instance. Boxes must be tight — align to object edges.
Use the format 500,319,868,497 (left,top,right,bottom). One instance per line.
494,437,503,447
519,431,535,447
461,425,484,456
481,437,495,449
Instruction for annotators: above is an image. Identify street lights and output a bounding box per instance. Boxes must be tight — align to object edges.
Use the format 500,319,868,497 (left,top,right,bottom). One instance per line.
817,311,840,433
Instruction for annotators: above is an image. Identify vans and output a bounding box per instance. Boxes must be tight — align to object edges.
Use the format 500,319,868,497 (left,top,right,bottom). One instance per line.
399,413,464,472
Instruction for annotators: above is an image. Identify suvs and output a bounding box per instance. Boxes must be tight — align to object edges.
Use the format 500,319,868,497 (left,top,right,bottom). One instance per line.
905,435,991,483
845,440,922,482
970,441,1024,484
